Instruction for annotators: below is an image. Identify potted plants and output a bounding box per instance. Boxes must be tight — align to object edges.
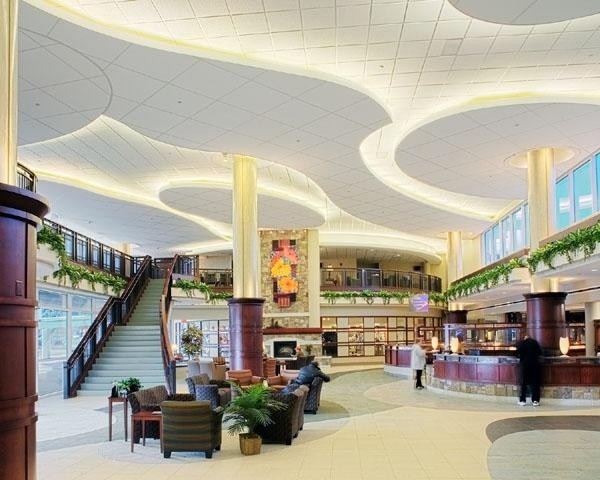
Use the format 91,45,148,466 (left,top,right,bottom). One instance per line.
115,376,145,396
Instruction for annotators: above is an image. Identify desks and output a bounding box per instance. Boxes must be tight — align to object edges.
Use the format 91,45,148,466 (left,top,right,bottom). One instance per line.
107,396,130,442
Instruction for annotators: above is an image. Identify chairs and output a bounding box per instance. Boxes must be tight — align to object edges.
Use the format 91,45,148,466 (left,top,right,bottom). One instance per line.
131,356,322,459
344,274,411,288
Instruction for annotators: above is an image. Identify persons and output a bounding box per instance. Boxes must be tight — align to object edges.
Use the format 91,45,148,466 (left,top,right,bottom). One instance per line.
410,337,430,390
515,334,543,407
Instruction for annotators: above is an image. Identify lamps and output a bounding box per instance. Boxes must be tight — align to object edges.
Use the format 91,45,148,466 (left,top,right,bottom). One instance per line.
450,337,458,356
559,336,570,358
431,336,440,352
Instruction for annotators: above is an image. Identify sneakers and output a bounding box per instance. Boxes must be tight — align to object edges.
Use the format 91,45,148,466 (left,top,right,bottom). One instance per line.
416,385,424,390
517,402,526,406
532,400,540,407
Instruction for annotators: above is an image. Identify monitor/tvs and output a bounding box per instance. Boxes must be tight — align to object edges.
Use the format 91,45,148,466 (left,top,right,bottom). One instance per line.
271,340,299,361
